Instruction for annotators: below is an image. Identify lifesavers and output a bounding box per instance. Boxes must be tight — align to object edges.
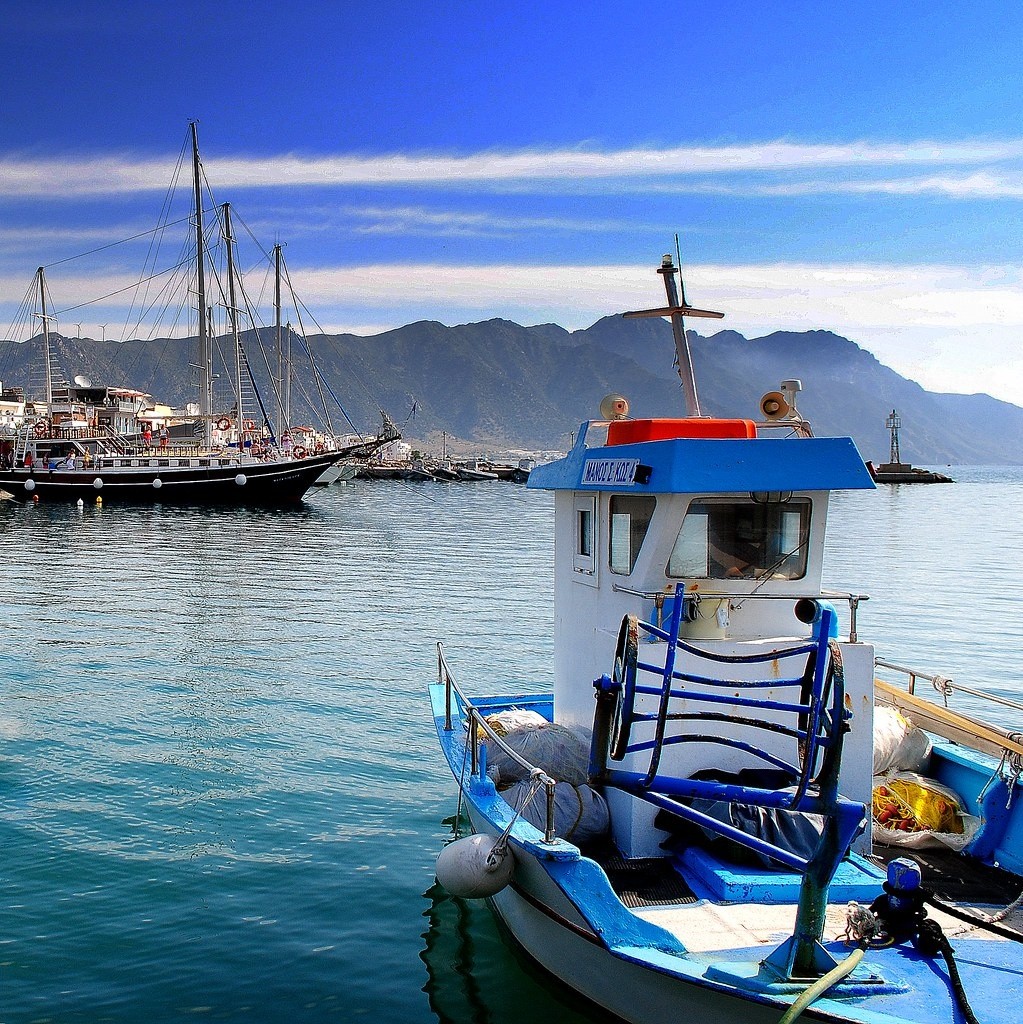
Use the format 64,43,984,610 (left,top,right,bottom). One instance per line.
217,417,230,431
243,419,254,432
34,421,46,435
293,444,307,459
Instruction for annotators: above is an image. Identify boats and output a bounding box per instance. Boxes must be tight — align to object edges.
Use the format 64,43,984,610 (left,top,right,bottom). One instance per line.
458,459,499,481
428,232,1023,1024
406,459,431,479
431,460,460,480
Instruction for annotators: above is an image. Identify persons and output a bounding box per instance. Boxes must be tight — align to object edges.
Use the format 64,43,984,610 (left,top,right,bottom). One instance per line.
8,451,13,470
93,451,99,471
281,430,293,457
159,424,169,453
144,425,152,453
42,452,51,469
84,446,93,471
251,437,277,463
66,448,75,470
24,451,33,468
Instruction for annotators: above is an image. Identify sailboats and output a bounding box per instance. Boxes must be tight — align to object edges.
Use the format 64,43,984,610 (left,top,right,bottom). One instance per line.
0,116,403,510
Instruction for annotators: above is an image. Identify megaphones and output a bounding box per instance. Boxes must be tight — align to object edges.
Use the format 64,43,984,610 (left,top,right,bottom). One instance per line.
760,391,789,420
600,393,629,420
